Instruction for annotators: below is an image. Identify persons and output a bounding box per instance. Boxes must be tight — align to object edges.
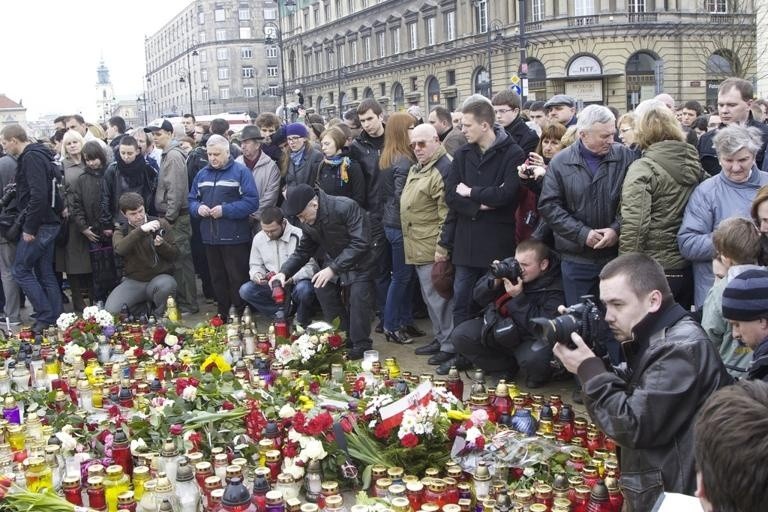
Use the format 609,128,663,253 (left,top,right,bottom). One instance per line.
694,379,768,512
1,78,768,403
552,252,729,512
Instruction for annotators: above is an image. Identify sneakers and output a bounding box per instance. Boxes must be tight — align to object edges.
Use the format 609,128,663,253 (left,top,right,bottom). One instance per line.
0,293,69,332
574,386,583,403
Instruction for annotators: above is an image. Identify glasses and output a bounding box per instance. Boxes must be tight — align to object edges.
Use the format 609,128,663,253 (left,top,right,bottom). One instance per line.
409,140,425,149
360,117,372,122
530,116,541,118
494,109,512,112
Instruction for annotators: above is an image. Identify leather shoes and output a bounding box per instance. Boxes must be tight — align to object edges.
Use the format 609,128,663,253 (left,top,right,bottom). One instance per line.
346,319,548,389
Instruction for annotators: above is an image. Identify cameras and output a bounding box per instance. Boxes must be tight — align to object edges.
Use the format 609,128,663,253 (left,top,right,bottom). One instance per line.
488,258,523,282
152,228,165,239
523,167,534,176
528,294,603,353
525,209,538,228
290,89,305,116
0,182,16,209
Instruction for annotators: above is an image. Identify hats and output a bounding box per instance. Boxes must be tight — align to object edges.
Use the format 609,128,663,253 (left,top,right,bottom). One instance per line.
722,269,768,321
286,183,315,215
144,119,173,130
236,126,264,142
544,95,575,107
285,124,308,136
432,261,455,299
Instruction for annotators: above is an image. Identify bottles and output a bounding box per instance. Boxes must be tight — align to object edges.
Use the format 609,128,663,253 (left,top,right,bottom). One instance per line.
0,295,626,512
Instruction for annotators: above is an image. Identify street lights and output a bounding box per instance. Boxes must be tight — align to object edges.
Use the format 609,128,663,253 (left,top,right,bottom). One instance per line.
178,39,199,117
487,19,504,100
264,0,295,125
134,76,153,126
105,94,115,119
